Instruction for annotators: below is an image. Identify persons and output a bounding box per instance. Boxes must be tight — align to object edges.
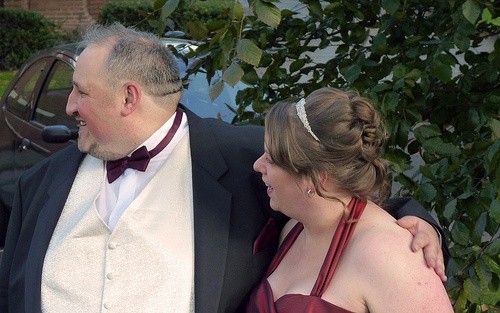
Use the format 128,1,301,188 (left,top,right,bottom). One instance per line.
0,21,451,313
247,88,455,313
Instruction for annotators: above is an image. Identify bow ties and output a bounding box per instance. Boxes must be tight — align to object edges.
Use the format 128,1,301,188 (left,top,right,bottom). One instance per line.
105,105,183,184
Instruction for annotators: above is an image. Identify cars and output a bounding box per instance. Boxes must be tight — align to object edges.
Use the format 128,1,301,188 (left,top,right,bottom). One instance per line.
0,38,267,245
